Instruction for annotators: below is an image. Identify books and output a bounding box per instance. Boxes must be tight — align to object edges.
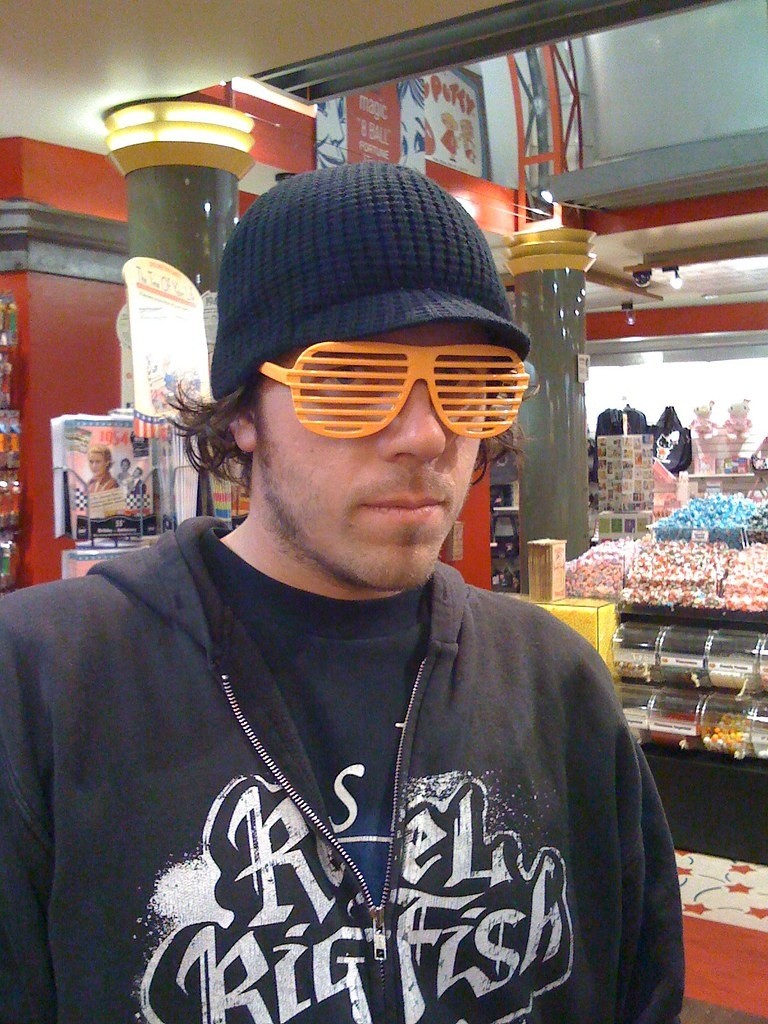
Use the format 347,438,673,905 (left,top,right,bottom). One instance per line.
49,407,252,583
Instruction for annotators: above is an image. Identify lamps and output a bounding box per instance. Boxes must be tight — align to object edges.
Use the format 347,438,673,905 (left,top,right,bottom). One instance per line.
632,270,652,288
621,302,636,327
662,266,683,289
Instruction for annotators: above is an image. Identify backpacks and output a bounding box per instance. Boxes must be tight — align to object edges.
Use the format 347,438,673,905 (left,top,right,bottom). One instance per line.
591,404,648,483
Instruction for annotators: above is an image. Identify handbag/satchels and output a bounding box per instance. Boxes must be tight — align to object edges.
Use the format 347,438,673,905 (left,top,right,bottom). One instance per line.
648,405,692,472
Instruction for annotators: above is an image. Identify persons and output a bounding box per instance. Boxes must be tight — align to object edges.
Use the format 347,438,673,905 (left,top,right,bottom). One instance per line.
117,458,131,481
1,162,687,1024
130,466,147,494
86,443,118,493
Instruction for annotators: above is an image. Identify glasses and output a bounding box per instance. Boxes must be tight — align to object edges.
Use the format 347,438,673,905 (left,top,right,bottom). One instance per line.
258,338,530,438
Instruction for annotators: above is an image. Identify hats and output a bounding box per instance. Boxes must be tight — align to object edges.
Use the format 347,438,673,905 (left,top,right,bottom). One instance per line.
210,165,533,402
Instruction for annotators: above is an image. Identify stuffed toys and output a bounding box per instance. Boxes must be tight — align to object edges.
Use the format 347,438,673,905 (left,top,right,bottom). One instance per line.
723,399,752,443
688,399,722,441
